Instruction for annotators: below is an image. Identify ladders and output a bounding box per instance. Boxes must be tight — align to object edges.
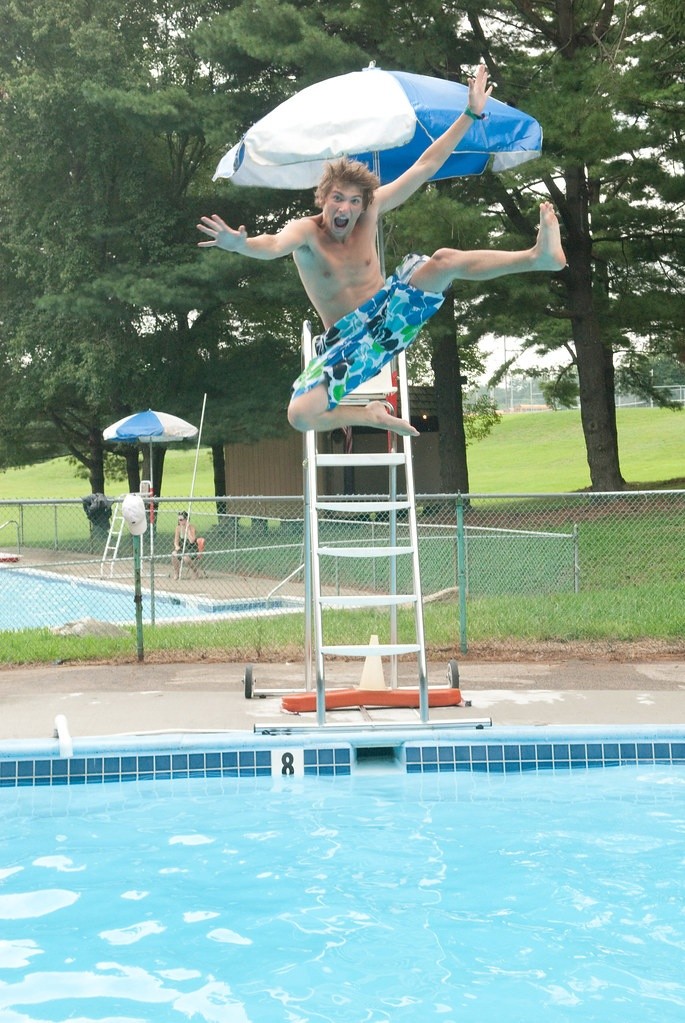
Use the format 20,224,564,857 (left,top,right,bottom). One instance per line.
251,318,494,734
86,480,173,580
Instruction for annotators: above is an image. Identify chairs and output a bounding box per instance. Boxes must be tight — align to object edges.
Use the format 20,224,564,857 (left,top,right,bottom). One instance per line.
183,538,208,579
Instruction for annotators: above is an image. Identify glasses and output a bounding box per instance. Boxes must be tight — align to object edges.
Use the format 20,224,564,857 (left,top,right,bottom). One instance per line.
178,518,185,522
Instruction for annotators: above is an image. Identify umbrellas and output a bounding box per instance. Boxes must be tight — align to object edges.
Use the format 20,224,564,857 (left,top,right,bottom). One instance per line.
211,60,543,278
102,408,199,553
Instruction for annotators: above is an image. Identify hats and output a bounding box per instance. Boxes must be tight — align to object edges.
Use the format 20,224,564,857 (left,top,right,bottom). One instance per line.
121,493,147,536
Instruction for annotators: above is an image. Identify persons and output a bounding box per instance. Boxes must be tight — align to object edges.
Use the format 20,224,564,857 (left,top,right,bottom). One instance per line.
172,511,199,580
196,66,567,436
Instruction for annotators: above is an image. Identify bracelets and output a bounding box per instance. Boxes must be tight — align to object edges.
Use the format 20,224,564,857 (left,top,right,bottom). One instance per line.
464,105,491,121
